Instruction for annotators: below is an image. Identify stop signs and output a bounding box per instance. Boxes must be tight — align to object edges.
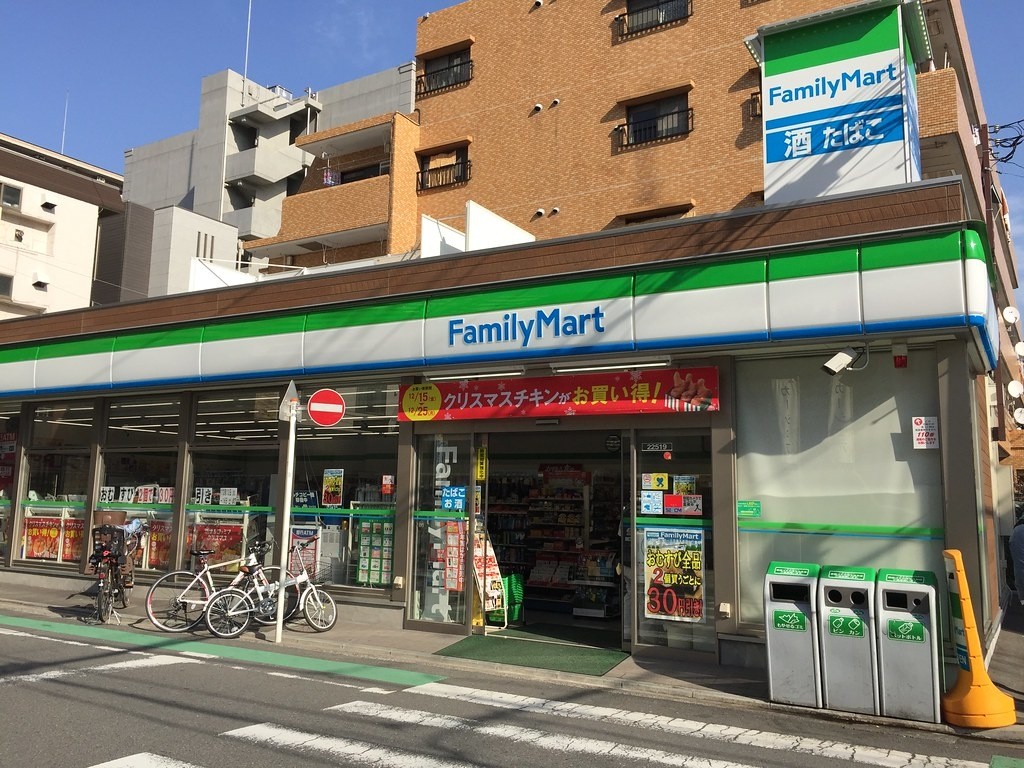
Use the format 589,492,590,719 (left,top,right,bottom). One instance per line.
306,388,346,428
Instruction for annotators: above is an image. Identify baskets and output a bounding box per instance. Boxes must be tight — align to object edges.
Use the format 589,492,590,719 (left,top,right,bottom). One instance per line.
303,559,333,584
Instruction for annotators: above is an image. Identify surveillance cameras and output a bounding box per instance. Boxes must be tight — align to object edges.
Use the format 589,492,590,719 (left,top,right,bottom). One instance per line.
820,346,860,376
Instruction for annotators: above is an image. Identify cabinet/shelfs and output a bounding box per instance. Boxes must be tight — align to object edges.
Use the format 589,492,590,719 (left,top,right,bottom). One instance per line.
27,453,620,617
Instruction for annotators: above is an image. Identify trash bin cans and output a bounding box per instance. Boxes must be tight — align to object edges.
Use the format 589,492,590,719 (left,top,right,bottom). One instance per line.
874,567,944,724
816,566,880,716
761,561,823,709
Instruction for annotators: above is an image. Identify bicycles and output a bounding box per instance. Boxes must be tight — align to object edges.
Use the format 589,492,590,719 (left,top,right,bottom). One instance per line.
88,521,147,623
203,534,337,639
145,535,300,634
